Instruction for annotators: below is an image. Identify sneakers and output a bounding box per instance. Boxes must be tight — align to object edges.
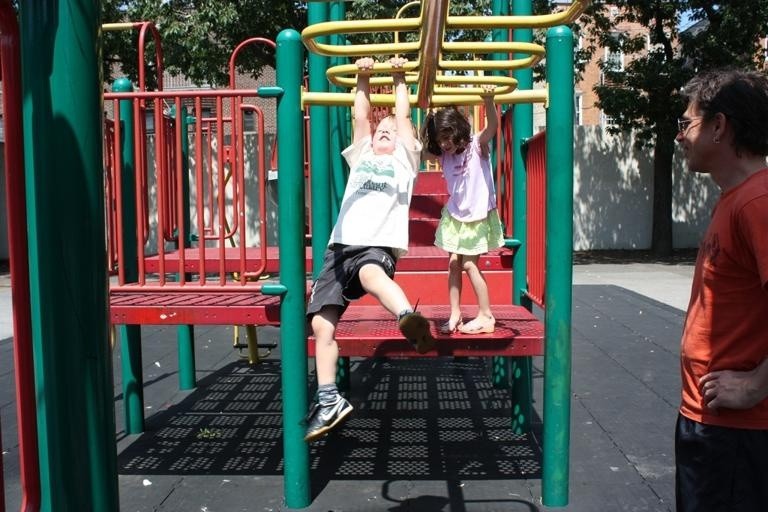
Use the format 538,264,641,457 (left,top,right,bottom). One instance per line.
304,393,354,442
398,310,436,354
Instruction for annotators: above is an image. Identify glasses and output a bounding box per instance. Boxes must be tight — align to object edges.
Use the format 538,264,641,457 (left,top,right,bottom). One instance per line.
677,117,707,133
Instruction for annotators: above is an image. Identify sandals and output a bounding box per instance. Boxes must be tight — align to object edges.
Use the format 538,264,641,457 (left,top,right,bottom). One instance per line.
459,319,495,334
440,312,463,334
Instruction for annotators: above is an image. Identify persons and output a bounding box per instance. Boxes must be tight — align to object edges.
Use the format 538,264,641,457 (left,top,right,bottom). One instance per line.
420,83,499,336
303,57,436,444
674,68,768,511
